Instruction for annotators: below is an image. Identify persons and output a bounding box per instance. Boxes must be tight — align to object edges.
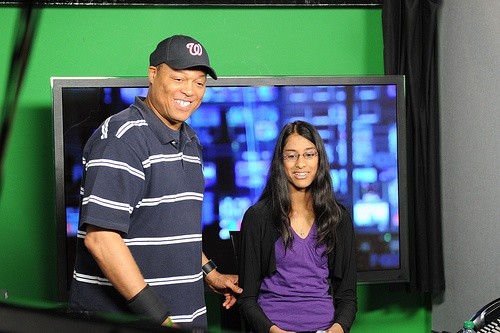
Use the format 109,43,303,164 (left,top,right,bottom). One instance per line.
71,35,242,328
236,119,359,333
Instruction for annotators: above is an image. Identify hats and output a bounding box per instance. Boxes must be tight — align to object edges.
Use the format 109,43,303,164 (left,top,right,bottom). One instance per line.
149,35,218,80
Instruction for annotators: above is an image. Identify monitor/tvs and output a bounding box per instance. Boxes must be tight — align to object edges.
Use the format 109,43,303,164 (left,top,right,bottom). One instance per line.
50,75,411,287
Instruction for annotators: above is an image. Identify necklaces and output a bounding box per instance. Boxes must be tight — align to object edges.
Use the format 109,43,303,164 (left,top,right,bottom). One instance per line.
292,219,311,237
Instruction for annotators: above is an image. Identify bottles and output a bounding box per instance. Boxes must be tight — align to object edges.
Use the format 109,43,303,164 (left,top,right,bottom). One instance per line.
463,321,476,333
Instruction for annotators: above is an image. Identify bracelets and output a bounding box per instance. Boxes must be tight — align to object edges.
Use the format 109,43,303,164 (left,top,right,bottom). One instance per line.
201,259,217,280
165,318,173,327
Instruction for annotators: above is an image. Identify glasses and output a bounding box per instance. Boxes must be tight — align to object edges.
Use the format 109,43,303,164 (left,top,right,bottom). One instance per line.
283,150,318,162
